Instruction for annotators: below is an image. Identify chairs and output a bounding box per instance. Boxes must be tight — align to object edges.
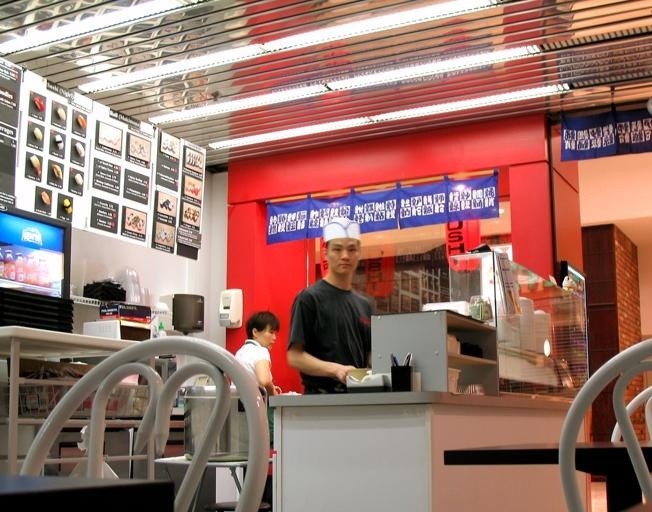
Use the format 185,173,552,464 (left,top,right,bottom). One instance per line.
18,335,270,512
558,337,651,512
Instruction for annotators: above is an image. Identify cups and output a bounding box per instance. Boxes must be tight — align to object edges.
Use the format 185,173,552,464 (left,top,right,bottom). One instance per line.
390,365,412,393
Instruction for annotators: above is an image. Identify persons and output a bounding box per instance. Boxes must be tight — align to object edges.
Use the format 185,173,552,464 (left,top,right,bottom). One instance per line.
228,311,283,504
286,217,375,394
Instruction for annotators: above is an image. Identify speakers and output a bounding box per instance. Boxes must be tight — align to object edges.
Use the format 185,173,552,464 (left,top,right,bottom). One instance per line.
173,293,204,332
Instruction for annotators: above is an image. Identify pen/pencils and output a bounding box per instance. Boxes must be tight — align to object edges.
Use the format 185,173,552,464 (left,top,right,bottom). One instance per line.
391,353,412,366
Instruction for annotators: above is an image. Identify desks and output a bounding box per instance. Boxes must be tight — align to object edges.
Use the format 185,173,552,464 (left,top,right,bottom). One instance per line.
268,391,587,512
443,441,651,512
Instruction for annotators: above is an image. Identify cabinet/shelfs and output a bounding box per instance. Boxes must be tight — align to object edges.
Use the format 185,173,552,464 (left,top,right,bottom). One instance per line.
1,325,155,478
370,251,588,398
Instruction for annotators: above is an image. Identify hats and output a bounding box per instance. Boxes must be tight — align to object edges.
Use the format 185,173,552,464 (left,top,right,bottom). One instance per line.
323,215,362,244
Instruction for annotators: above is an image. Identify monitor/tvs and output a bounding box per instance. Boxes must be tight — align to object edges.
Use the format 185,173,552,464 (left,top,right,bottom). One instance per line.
0,203,72,299
